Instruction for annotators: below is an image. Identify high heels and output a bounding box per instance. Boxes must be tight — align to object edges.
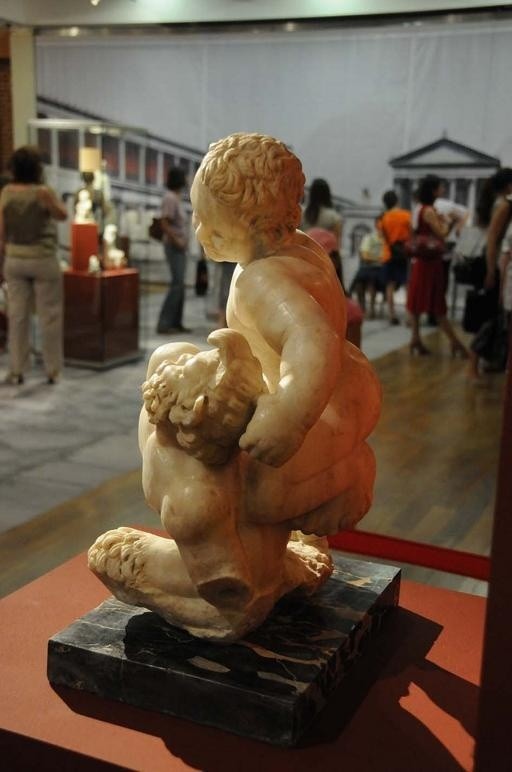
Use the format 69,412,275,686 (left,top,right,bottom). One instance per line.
410,343,431,355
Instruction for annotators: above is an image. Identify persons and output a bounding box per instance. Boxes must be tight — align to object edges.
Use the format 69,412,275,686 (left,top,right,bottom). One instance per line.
347,165,512,359
190,133,384,589
71,170,104,218
155,163,193,334
218,261,238,331
302,178,345,293
1,146,69,385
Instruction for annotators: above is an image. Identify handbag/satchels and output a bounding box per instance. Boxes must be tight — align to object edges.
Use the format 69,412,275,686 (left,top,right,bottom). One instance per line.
463,289,498,334
149,217,167,239
453,254,501,288
472,310,508,371
413,207,444,258
195,259,209,298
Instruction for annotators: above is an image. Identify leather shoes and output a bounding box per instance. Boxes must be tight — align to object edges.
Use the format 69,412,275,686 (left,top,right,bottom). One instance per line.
451,346,470,361
47,369,63,384
175,322,192,334
5,373,22,384
158,323,182,334
359,306,402,324
